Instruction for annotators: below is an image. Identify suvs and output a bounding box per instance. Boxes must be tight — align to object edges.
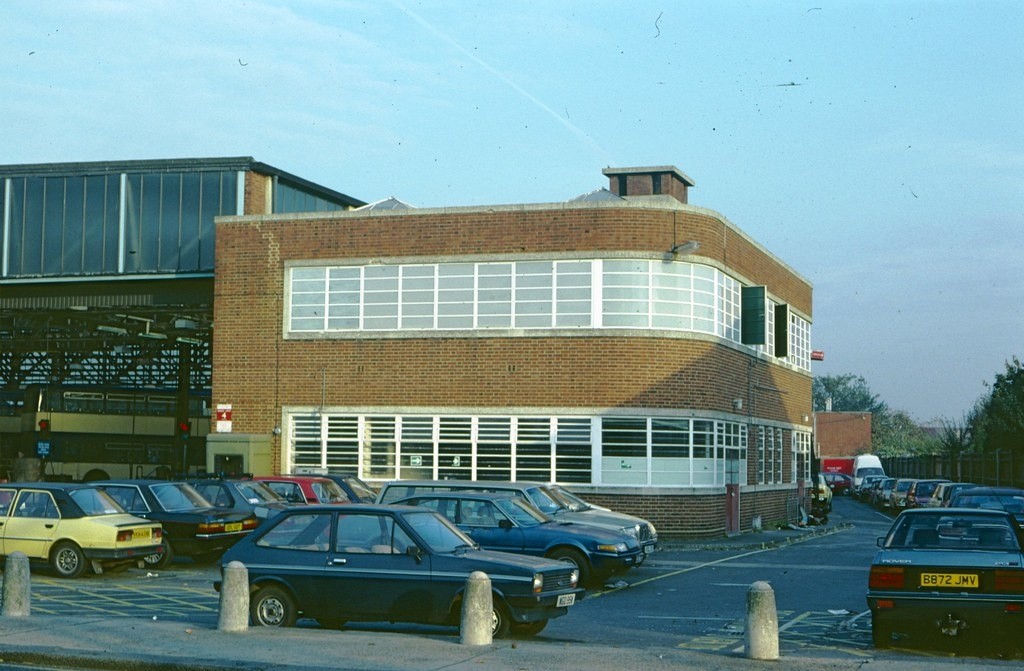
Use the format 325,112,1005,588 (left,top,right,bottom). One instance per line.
319,478,659,590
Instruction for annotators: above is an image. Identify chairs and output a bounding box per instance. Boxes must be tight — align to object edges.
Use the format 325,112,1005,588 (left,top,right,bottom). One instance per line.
462,506,495,524
345,545,401,554
978,528,1007,546
913,529,940,547
30,505,46,517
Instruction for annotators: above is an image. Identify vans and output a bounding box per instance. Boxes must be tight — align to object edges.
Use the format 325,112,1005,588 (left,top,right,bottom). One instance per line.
851,454,886,498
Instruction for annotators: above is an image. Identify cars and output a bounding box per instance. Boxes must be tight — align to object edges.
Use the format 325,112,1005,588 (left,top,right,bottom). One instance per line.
0,482,164,579
810,475,835,512
865,507,1024,650
857,474,1024,544
67,479,260,570
819,472,852,496
197,471,378,505
212,504,580,641
180,479,291,513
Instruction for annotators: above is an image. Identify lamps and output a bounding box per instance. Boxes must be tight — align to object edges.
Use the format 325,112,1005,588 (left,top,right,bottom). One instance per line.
176,336,202,344
733,397,744,411
802,413,808,421
97,326,127,334
671,240,701,255
138,332,168,339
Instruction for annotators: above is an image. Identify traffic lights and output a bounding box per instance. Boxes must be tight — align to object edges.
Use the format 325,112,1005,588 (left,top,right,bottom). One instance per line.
179,421,191,441
39,419,50,442
